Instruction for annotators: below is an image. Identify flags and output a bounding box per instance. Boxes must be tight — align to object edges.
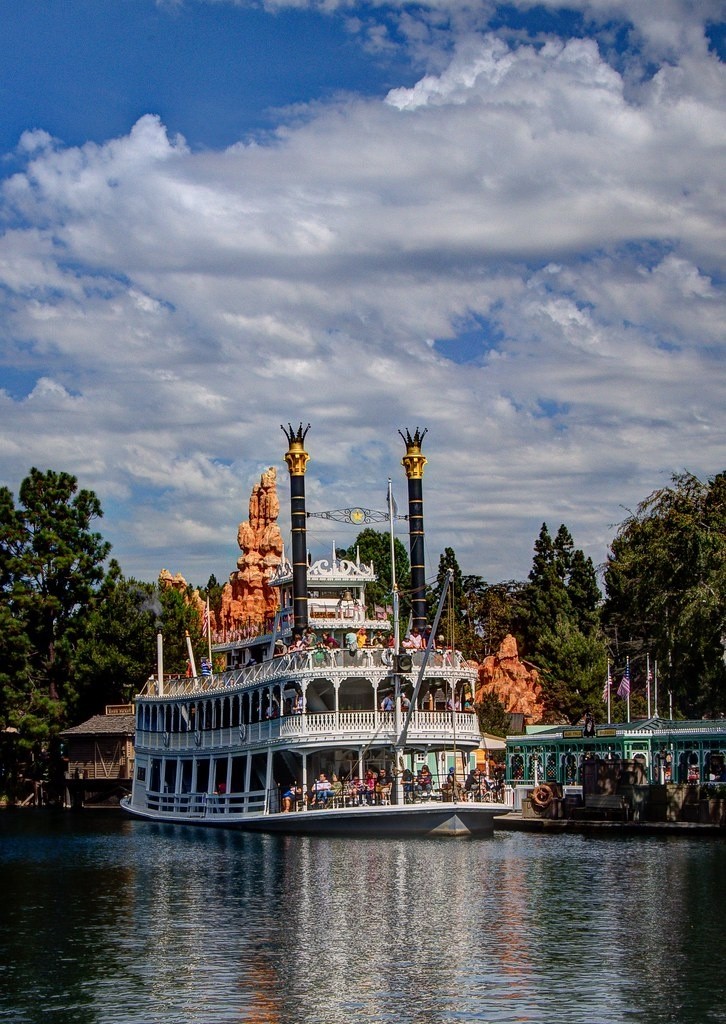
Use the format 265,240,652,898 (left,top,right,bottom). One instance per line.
603,666,612,703
646,665,652,700
617,661,630,699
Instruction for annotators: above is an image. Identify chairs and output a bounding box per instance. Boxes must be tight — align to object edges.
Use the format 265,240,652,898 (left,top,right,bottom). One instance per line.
295,782,502,812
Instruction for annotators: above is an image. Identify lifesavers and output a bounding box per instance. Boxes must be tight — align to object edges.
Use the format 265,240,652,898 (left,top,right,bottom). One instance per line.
380,647,396,666
162,732,170,745
238,724,246,740
194,731,202,745
533,784,553,806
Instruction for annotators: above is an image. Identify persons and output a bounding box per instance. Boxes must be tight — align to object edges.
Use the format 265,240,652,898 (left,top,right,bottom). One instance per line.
401,693,411,708
273,626,446,658
464,698,475,713
261,691,306,718
247,657,257,667
446,695,460,710
382,690,394,710
280,766,506,813
213,782,234,793
203,657,210,675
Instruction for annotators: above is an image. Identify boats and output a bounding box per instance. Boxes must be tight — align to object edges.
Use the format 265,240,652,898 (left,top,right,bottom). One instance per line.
120,423,515,836
492,717,725,830
